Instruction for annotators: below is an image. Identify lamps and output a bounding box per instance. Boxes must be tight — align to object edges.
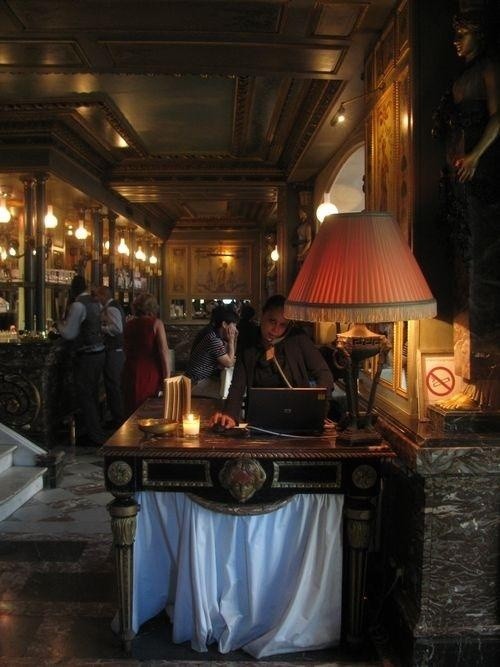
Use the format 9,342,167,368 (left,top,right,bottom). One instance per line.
283,212,438,447
271,245,280,261
0,197,158,264
330,87,386,128
316,192,338,224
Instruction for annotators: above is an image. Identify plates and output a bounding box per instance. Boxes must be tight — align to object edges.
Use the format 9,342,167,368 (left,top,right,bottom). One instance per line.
138,418,179,439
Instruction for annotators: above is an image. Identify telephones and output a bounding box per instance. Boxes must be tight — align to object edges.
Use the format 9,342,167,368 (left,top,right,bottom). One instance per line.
272,320,295,345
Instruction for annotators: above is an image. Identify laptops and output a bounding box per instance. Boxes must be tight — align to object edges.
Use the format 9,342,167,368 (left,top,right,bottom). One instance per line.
244,387,328,437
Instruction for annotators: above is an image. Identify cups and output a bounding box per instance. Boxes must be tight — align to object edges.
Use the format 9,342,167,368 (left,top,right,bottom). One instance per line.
183,413,200,439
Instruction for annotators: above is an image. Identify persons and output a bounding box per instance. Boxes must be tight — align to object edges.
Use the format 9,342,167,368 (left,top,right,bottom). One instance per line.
237,306,253,329
122,291,171,404
191,305,224,349
186,308,240,397
51,274,109,445
93,286,134,429
204,295,336,428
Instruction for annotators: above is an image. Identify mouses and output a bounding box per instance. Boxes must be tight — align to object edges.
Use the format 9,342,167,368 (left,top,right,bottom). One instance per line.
211,422,225,432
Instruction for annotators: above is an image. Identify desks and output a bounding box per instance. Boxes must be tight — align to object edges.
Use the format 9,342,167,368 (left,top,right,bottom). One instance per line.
96,396,398,662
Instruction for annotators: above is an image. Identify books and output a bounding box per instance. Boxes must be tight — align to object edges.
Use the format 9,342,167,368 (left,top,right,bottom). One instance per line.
163,373,192,421
